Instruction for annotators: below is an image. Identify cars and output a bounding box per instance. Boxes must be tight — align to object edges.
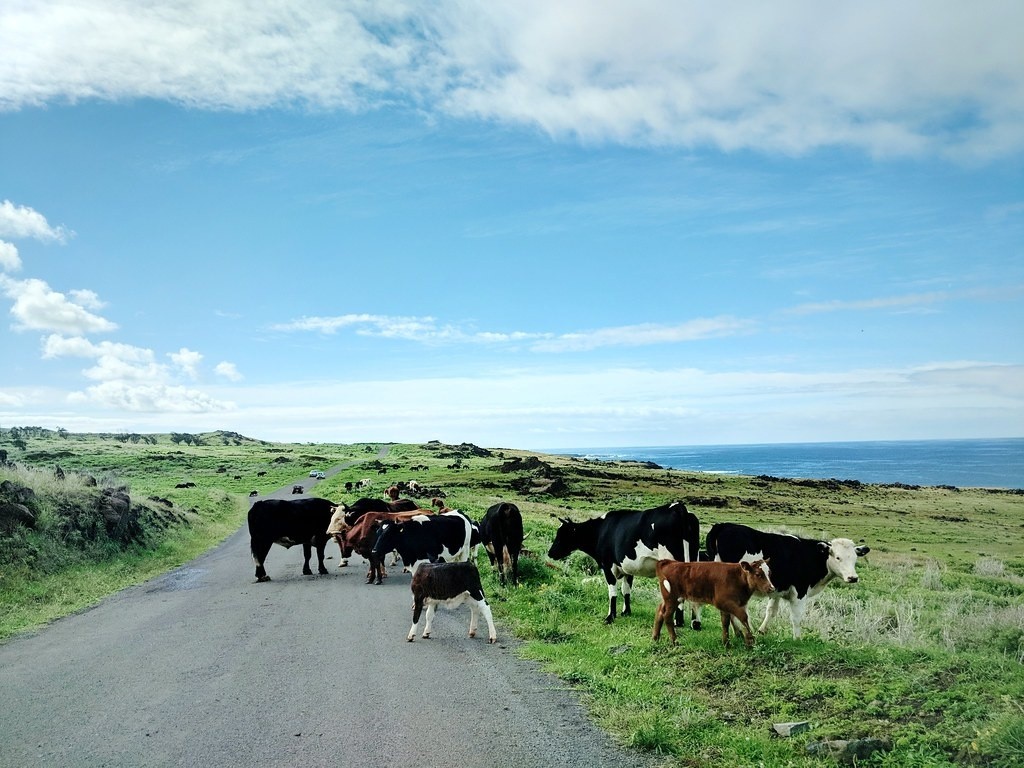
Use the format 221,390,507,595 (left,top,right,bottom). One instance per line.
309,470,317,477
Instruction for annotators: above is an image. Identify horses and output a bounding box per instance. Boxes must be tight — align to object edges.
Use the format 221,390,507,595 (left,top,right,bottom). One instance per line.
548,500,870,650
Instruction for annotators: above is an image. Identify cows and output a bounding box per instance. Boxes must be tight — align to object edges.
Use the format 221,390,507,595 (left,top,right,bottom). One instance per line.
344,463,469,500
173,472,267,497
246,497,523,644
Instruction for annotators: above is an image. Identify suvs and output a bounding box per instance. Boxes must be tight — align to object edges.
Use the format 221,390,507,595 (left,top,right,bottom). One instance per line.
292,485,304,495
316,472,326,480
